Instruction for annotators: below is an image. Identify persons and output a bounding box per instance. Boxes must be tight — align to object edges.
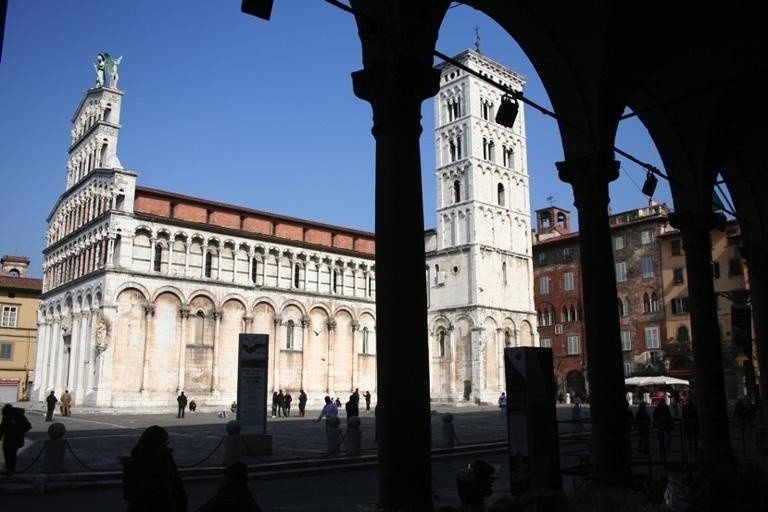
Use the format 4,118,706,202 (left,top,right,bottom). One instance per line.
316,388,359,437
0,404,32,473
559,388,575,403
272,389,307,418
190,400,196,412
45,390,71,421
499,393,506,416
177,392,186,418
195,462,262,512
363,391,370,410
625,397,697,448
120,425,189,512
733,399,757,424
